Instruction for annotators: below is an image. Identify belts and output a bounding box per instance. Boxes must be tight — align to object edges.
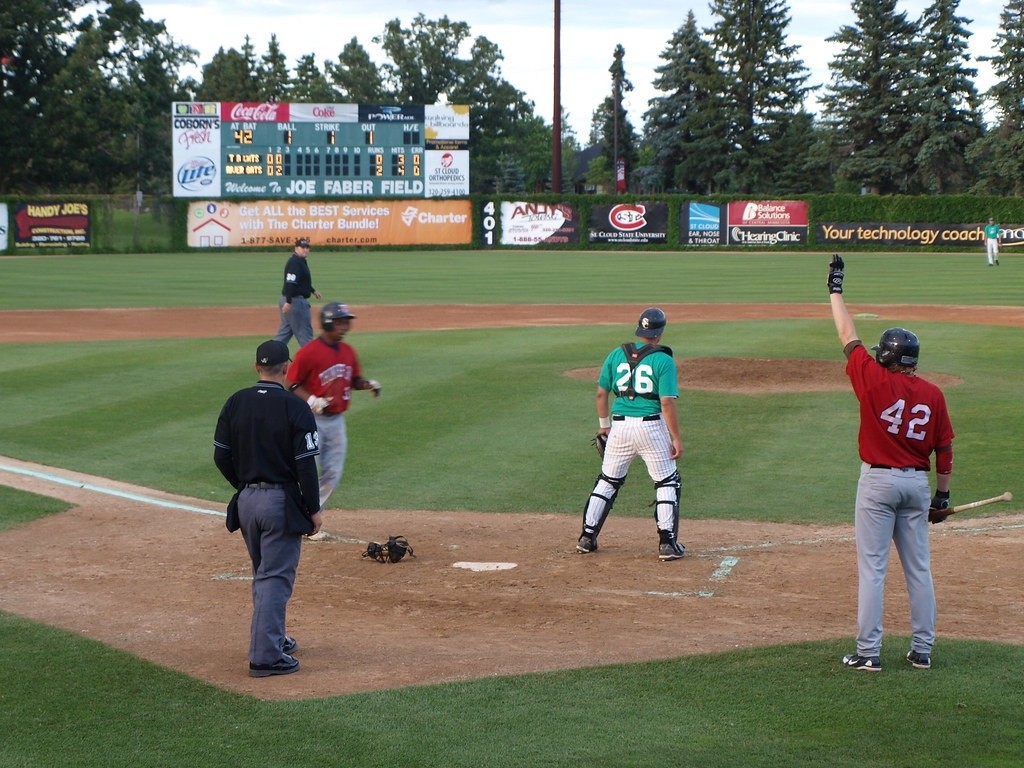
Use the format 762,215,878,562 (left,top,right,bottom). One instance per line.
246,483,284,489
613,414,660,421
870,463,927,471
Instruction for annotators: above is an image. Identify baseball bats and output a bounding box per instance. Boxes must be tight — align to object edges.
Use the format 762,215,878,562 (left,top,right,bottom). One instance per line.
928,492,1013,522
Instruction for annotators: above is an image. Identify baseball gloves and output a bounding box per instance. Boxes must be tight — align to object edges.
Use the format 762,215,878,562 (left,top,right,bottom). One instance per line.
594,434,608,459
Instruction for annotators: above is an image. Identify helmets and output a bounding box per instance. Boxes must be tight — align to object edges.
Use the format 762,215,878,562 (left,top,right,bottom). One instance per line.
871,327,919,372
319,302,355,330
635,307,666,338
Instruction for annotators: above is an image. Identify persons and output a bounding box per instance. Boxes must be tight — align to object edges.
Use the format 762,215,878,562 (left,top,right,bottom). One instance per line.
284,301,381,540
826,253,955,671
275,239,321,348
576,307,686,560
984,218,1002,266
213,339,322,677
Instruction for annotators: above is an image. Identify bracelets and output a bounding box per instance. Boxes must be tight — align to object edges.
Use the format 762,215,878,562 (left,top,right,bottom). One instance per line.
307,394,317,406
599,417,610,428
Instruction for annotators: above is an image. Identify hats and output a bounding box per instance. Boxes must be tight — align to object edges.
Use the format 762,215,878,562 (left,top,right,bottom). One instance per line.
989,218,994,221
255,339,293,366
295,239,310,246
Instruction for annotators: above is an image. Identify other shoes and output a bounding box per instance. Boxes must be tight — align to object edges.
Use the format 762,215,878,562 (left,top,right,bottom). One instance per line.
989,264,993,266
995,259,999,265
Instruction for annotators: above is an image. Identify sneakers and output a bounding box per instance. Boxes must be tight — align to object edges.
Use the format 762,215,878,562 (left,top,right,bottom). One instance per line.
842,652,881,671
658,542,685,559
249,652,299,677
282,635,296,655
576,536,597,553
906,649,930,669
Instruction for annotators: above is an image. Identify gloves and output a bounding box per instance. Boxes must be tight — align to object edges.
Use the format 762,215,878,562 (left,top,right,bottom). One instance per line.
368,379,382,396
827,253,844,293
928,488,950,524
307,394,333,413
